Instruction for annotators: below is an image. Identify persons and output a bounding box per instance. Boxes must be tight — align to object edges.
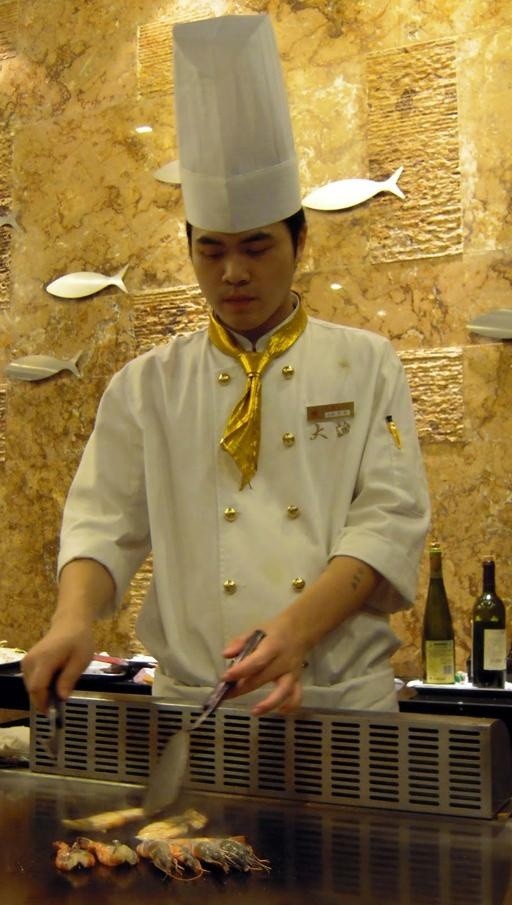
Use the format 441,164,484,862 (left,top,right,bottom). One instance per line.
20,14,433,718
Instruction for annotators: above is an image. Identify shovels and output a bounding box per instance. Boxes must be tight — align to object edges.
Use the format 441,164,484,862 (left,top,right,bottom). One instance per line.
139,628,271,818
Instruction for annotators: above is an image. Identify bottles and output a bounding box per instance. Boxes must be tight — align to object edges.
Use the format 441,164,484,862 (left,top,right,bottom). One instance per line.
470,553,507,688
423,541,456,685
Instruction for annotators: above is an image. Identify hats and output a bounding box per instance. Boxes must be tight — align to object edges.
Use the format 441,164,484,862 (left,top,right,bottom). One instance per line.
173,14,300,235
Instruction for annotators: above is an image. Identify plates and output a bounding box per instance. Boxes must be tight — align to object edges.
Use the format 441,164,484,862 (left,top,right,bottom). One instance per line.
0,648,28,666
